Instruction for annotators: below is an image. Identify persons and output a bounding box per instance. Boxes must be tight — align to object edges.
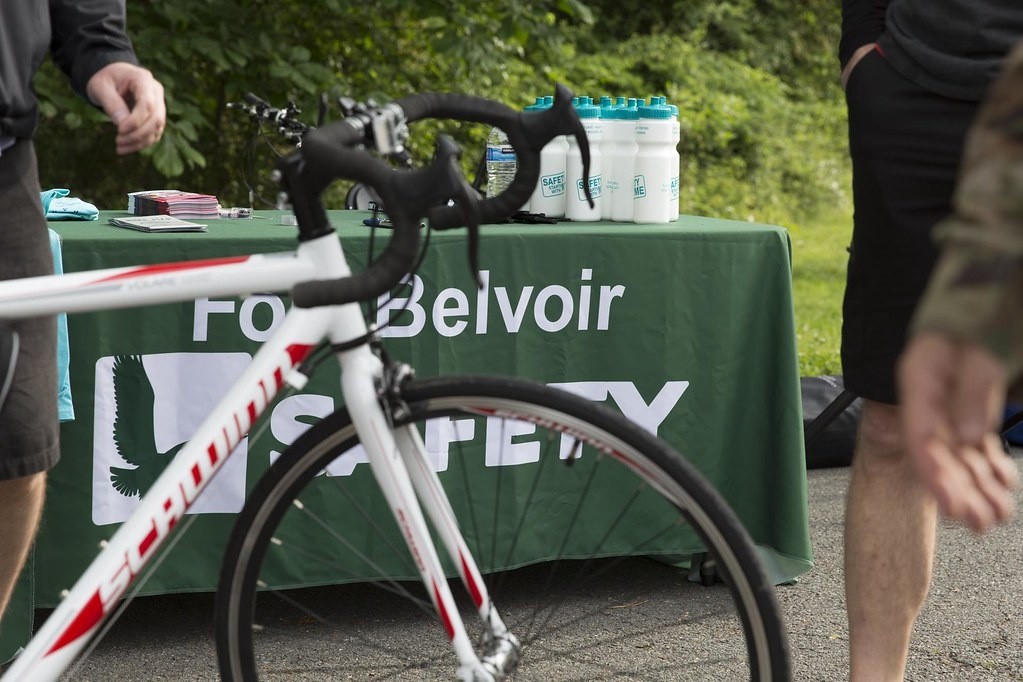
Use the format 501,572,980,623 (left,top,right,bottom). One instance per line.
895,50,1023,532
838,1,1023,682
0,0,166,619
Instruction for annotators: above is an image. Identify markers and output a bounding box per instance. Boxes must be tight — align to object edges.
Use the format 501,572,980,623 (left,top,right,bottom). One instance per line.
363,216,426,230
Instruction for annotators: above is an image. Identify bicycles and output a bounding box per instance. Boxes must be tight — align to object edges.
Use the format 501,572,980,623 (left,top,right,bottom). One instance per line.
0,85,791,682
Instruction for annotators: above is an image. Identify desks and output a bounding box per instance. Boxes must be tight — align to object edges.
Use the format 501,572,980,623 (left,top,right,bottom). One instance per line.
0,207,815,664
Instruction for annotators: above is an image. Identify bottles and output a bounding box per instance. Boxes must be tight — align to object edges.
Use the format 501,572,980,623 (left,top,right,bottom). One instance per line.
485,126,519,199
523,95,681,224
